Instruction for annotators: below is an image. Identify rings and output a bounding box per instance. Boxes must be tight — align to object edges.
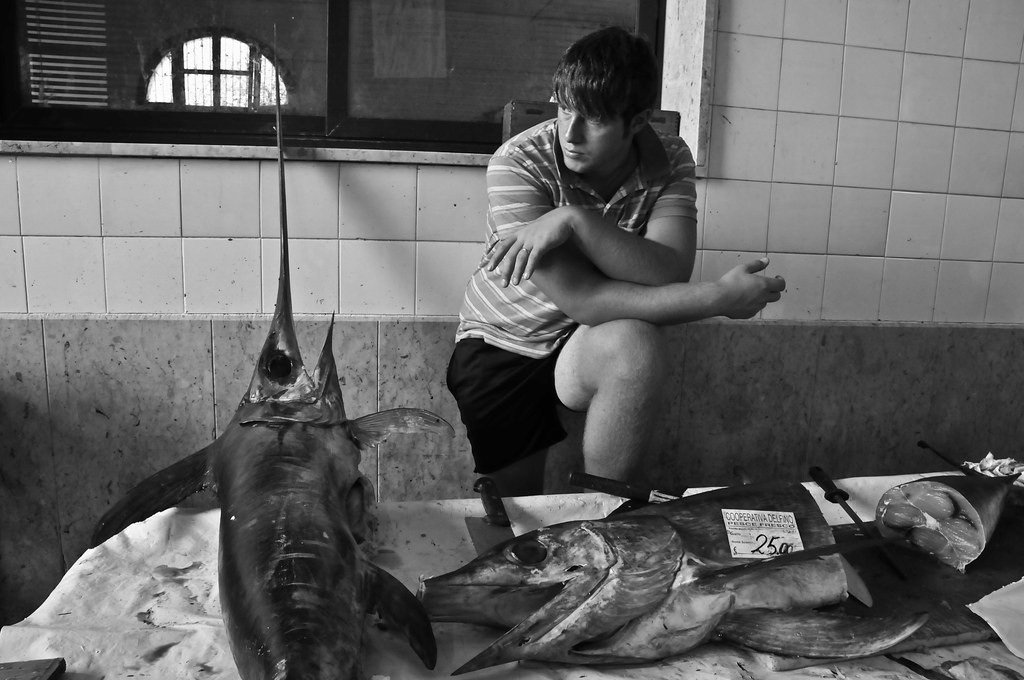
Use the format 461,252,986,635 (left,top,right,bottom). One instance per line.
522,248,530,254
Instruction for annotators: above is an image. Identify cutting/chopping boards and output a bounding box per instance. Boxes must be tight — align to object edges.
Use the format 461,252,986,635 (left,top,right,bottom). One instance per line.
749,520,1024,673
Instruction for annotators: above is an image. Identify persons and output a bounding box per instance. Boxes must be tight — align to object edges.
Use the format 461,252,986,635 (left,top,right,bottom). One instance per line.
443,27,786,497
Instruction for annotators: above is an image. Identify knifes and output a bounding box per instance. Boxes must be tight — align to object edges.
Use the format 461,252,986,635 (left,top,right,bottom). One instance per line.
838,552,874,608
464,475,516,557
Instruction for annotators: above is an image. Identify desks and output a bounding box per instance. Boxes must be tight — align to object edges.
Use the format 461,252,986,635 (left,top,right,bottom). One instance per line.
0,468,1024,680
